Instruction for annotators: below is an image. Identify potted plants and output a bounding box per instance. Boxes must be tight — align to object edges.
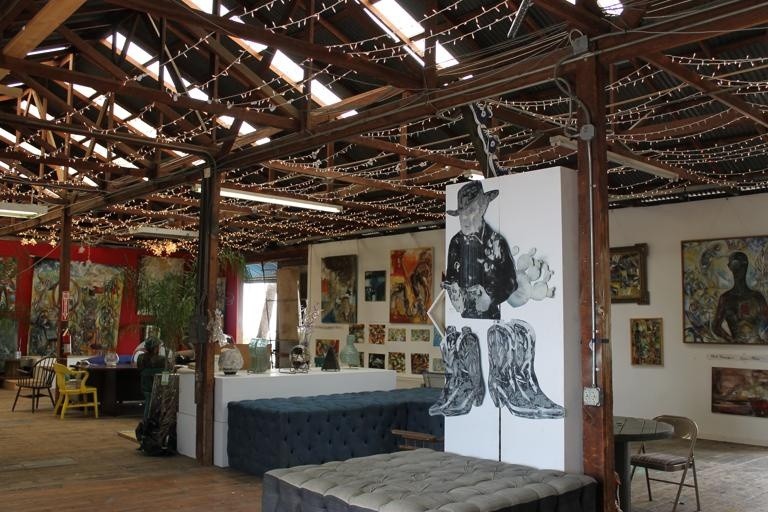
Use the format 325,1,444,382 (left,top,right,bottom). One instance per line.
135,248,252,457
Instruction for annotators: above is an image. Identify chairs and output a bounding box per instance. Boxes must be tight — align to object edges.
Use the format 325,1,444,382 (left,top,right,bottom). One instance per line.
624,415,701,511
51,363,99,419
10,355,56,414
391,428,444,453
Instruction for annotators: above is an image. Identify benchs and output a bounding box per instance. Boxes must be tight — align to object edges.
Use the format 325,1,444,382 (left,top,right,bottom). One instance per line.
227,386,406,478
387,385,446,452
262,446,598,512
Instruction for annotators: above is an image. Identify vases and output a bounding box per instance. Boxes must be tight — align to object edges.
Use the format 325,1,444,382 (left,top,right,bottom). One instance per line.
104,348,121,367
338,334,360,366
289,344,311,373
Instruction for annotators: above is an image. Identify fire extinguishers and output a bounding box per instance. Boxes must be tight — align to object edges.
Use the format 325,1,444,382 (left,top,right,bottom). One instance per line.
60,326,72,356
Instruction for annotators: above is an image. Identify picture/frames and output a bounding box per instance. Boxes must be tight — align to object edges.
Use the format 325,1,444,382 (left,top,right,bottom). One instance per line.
628,317,663,366
678,234,768,348
609,240,649,306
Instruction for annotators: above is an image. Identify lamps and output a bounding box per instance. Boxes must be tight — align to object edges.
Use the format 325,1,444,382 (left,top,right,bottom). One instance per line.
130,226,198,241
0,183,49,220
189,183,344,214
547,135,681,183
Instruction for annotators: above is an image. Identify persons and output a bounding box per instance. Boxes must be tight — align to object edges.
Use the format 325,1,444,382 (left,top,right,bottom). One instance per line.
710,251,767,343
441,181,521,321
136,337,171,375
321,262,356,323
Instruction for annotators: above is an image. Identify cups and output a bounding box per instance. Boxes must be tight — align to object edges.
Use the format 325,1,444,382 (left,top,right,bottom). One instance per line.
14,351,21,360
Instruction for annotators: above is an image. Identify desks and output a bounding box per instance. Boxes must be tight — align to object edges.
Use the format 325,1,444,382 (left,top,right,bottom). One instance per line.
611,415,675,512
68,365,142,414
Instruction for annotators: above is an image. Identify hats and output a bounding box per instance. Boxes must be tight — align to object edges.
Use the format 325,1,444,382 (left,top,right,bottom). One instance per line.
446,180,499,216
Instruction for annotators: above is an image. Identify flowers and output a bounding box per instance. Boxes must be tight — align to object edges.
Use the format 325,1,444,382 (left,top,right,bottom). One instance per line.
295,296,320,345
